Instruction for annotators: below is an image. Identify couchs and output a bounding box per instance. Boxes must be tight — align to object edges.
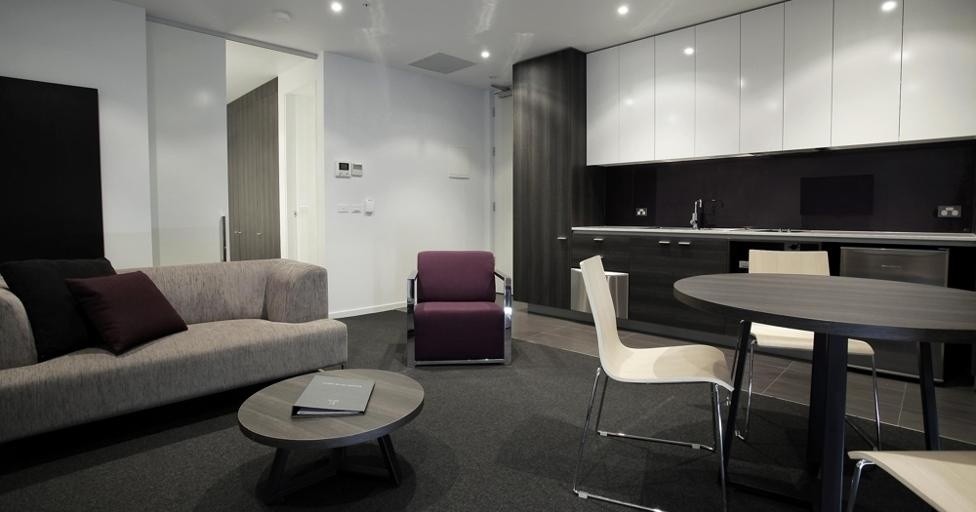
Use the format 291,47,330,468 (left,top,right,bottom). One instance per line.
407,251,512,370
0,258,349,457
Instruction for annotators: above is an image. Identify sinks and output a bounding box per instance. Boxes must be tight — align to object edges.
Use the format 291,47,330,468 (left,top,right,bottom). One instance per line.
755,228,802,233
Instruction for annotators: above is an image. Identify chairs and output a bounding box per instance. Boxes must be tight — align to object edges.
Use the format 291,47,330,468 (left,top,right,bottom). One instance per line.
572,255,735,511
728,249,882,452
844,448,976,511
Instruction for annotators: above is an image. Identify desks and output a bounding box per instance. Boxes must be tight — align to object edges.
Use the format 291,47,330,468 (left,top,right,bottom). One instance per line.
673,272,976,510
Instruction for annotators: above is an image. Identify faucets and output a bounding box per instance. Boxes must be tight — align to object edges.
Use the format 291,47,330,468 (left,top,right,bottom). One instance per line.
689,198,702,230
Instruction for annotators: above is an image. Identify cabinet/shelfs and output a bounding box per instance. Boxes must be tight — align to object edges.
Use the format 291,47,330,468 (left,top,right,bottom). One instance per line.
840,225,963,386
570,227,738,344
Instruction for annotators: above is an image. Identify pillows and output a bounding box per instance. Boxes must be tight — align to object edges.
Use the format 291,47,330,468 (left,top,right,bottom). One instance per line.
66,270,187,357
0,257,124,363
